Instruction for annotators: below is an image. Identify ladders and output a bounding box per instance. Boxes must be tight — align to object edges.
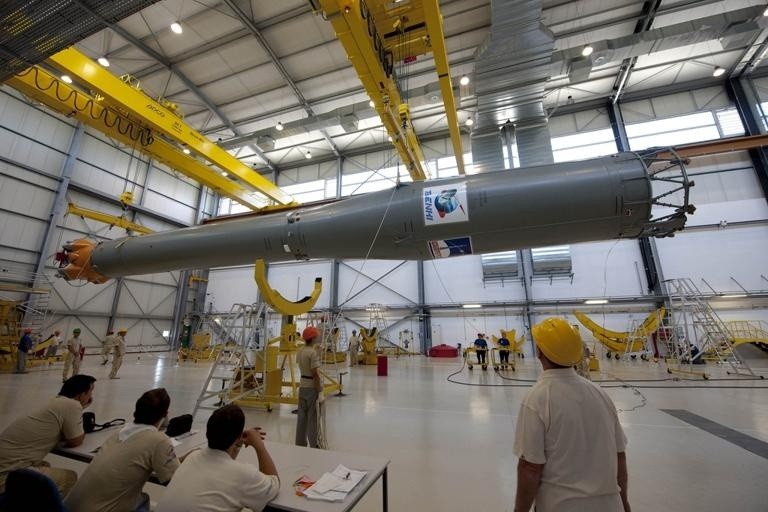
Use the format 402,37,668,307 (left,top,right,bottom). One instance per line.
321,311,340,385
324,311,348,351
660,278,766,380
369,303,393,347
623,318,654,360
190,300,267,420
656,307,690,364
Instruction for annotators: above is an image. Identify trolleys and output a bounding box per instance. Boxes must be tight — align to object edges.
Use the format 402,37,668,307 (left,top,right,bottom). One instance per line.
462,347,490,370
491,348,517,372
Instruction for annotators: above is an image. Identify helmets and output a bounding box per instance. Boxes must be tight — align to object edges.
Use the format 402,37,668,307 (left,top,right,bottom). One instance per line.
73,328,81,334
303,327,319,340
24,328,32,334
531,317,584,366
119,327,128,332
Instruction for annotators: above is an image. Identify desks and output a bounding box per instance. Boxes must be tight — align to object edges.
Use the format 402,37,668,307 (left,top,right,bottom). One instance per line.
49,421,392,512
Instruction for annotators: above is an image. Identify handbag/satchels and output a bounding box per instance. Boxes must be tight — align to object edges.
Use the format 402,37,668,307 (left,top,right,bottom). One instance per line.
83,412,125,434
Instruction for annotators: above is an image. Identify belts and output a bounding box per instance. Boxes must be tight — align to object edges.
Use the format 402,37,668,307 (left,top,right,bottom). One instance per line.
301,375,313,380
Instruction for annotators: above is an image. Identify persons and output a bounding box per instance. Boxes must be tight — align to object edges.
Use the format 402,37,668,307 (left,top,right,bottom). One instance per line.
45,329,62,359
317,316,329,341
577,338,593,382
61,385,203,511
511,313,632,512
473,331,491,365
496,333,512,370
62,327,84,382
682,337,705,364
151,401,281,511
109,326,128,380
1,371,97,511
15,326,35,374
102,330,114,366
346,330,361,367
292,326,328,448
